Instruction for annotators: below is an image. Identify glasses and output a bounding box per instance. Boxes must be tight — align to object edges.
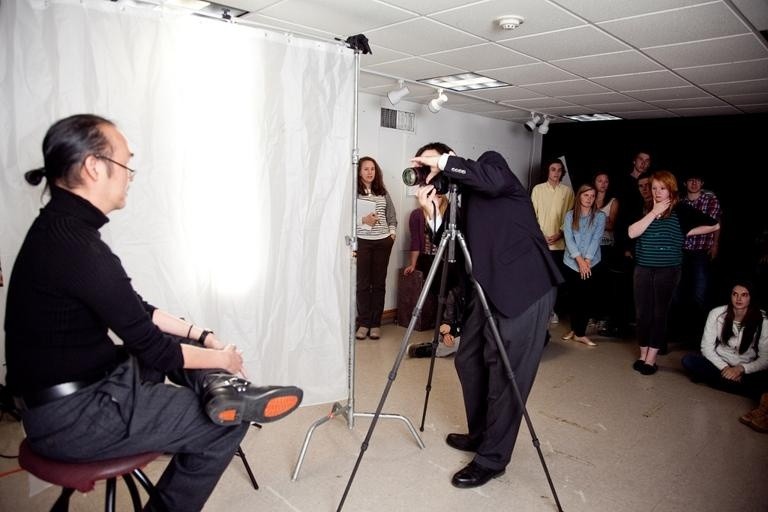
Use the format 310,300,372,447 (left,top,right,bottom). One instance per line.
80,153,138,183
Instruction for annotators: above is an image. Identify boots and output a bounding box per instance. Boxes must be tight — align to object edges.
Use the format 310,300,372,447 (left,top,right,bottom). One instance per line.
739,394,768,433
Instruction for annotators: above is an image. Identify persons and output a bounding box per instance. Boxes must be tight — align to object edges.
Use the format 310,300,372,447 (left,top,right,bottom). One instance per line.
561,183,608,347
636,170,655,212
627,169,720,376
2,112,304,512
739,377,767,433
356,155,397,339
660,171,723,354
409,142,565,489
681,279,767,399
592,171,620,337
531,158,576,324
403,182,451,319
405,269,474,359
615,151,654,336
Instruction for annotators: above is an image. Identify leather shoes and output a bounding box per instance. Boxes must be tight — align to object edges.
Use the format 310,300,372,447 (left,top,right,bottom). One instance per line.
451,461,505,488
201,372,303,427
447,434,477,452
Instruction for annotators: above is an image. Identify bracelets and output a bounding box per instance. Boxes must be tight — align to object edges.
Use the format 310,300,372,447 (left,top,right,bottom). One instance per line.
187,324,193,339
198,328,214,345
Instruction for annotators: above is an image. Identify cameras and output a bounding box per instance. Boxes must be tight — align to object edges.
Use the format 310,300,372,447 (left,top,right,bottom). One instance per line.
401,166,449,196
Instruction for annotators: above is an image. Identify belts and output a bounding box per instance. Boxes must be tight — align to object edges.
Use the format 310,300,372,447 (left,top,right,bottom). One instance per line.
25,368,106,411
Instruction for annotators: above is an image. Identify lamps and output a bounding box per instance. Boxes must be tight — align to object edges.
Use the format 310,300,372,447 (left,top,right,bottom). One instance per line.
385,78,551,136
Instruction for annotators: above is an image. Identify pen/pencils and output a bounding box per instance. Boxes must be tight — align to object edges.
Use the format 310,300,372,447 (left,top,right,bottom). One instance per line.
373,214,381,224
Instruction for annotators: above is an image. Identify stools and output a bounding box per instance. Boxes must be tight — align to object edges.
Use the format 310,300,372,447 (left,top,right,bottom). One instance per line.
18,435,159,512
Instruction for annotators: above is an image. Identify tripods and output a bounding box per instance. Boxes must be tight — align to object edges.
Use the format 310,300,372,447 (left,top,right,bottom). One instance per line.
336,190,563,512
289,50,426,484
